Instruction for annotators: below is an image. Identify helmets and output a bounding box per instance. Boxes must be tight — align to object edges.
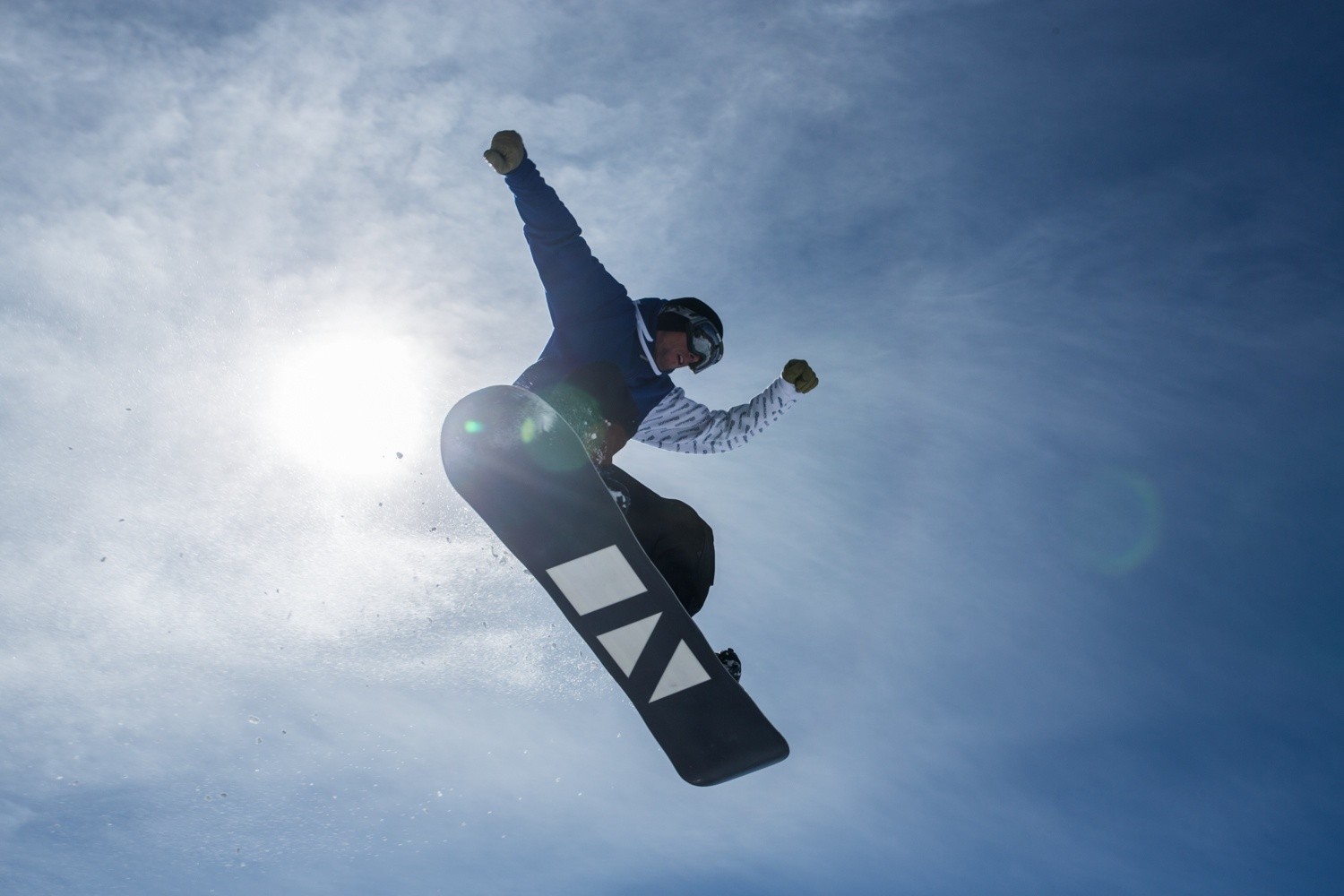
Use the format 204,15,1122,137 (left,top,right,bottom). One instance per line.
661,297,724,374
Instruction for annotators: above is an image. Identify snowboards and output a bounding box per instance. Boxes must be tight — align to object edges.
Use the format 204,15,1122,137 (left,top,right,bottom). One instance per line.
439,384,791,789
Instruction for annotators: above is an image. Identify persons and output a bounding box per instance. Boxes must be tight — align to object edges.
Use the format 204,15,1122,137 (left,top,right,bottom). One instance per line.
483,130,819,683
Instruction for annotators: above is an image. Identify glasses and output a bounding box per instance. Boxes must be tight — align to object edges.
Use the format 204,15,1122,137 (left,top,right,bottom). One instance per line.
687,325,714,371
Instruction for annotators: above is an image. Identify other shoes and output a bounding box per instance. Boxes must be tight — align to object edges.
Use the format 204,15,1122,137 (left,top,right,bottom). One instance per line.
717,647,742,681
603,476,631,517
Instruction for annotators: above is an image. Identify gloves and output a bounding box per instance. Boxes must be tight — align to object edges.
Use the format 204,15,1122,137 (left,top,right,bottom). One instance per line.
484,131,526,175
782,359,819,395
